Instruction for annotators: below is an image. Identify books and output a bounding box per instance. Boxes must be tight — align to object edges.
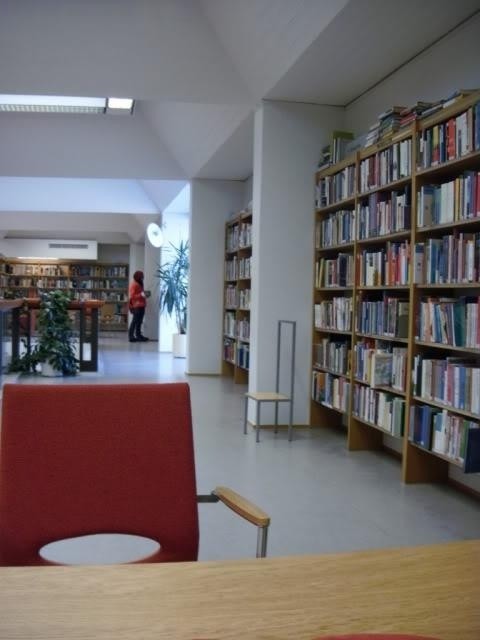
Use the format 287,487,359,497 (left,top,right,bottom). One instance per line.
0,262,129,325
222,199,253,369
312,88,480,473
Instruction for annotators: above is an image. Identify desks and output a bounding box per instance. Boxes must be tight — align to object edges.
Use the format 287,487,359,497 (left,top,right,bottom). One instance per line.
1,540,480,639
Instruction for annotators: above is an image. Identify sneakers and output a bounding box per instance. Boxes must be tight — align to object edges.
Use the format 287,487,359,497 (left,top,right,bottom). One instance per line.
129,335,148,341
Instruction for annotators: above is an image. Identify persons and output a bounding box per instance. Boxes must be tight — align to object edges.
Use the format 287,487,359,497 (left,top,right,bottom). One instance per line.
127,271,151,342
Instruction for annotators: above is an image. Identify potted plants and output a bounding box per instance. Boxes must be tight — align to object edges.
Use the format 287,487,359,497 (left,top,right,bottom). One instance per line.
152,235,188,357
4,288,78,379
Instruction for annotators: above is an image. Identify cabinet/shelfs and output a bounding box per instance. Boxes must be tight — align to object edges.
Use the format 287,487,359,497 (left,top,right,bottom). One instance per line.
0,254,128,331
223,209,252,387
310,90,479,484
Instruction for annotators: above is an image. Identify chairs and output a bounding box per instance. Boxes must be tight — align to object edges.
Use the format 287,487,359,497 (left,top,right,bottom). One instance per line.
2,380,269,565
245,320,295,443
